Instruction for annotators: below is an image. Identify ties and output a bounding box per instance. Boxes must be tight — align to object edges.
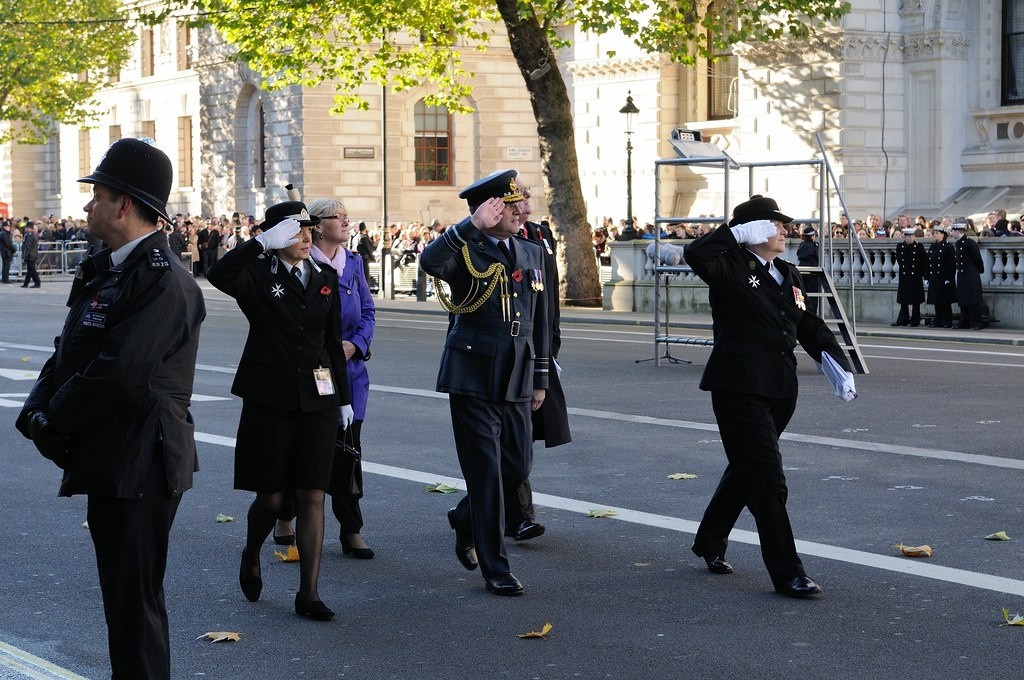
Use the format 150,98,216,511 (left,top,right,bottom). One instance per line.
498,239,514,269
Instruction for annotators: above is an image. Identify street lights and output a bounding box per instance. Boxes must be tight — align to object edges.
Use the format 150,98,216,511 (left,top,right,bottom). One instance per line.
618,88,640,238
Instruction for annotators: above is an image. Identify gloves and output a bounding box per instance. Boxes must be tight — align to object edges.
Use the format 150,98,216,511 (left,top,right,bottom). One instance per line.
340,404,355,430
835,372,856,402
729,219,777,245
25,410,70,462
256,218,301,251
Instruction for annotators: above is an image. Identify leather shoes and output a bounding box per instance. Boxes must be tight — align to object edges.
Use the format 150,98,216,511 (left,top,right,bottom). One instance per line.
447,507,478,572
692,544,734,574
780,574,821,599
513,520,544,543
486,573,525,595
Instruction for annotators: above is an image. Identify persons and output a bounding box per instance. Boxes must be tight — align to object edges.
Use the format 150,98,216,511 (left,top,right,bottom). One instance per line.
785,209,1024,331
155,211,265,279
15,138,205,680
272,199,375,558
684,195,855,596
206,200,353,618
418,168,572,595
0,214,110,288
345,219,449,298
589,213,723,266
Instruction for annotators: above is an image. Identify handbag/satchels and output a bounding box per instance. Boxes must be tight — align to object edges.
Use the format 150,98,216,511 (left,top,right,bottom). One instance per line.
326,418,364,499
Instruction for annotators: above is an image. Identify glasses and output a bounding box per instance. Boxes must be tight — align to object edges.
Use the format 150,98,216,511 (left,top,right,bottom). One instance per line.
836,231,843,234
318,214,349,222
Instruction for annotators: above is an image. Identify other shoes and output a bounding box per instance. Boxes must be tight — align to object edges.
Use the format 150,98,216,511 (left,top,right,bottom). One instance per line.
973,324,983,329
240,549,264,602
944,323,950,328
951,325,969,329
890,321,907,327
929,323,940,328
294,592,335,619
907,322,919,327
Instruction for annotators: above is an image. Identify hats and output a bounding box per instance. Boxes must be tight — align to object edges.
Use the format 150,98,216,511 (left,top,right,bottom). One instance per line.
729,197,793,223
950,220,969,231
803,228,817,236
459,169,526,202
902,228,918,235
77,138,175,225
257,202,321,228
933,225,946,233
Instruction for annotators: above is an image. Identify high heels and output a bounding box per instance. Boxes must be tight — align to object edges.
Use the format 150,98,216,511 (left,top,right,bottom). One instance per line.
270,522,295,544
339,532,375,559
